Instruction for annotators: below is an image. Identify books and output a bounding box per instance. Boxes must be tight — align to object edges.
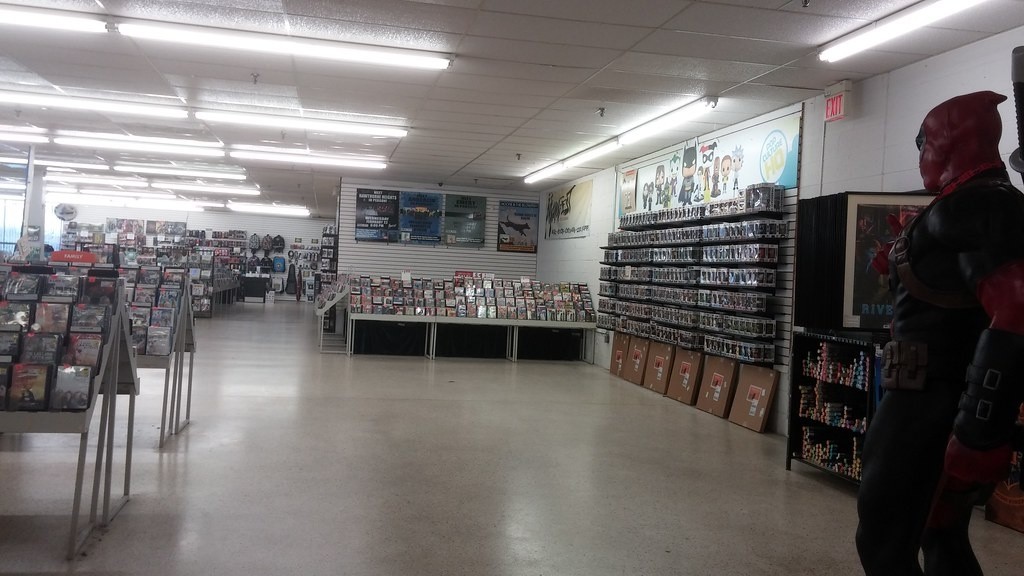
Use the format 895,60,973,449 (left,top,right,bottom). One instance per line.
350,269,596,322
118,264,185,355
190,267,212,312
0,271,117,411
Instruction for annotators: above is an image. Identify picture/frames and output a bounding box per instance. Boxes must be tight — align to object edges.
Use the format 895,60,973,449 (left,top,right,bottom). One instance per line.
793,191,941,333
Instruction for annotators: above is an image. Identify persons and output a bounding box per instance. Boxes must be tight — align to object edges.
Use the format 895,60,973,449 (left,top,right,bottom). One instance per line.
854,90,1024,576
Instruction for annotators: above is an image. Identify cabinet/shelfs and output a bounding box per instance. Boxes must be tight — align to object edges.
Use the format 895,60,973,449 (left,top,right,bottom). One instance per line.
596,210,789,370
320,234,338,334
785,331,887,485
0,224,240,560
312,276,351,354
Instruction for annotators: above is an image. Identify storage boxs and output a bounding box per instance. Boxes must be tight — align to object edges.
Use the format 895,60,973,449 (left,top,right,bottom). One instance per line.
666,346,705,406
596,183,788,363
643,342,676,394
610,332,631,377
696,355,739,418
728,364,780,433
623,335,651,386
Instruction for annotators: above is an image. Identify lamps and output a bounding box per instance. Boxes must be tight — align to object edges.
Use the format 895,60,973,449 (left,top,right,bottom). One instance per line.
0,3,457,72
0,88,411,138
523,96,719,185
816,0,987,63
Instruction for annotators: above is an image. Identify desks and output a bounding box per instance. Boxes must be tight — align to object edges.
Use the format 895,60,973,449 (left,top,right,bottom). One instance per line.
346,313,597,365
243,277,269,303
